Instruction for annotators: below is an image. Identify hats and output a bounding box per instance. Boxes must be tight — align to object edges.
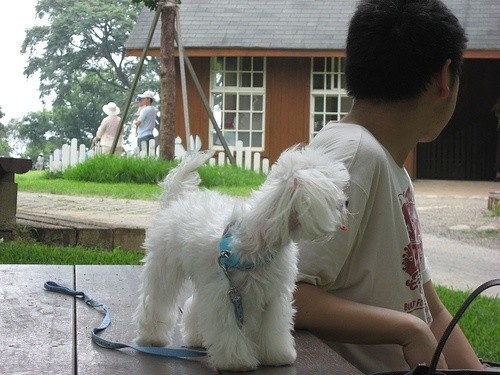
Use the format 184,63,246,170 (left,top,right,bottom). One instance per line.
102,102,120,116
138,91,154,100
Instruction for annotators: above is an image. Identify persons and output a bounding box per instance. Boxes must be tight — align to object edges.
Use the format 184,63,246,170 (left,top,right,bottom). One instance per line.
132,91,156,152
91,102,125,156
58,146,63,161
291,0,483,375
35,152,44,170
49,150,54,164
134,94,147,124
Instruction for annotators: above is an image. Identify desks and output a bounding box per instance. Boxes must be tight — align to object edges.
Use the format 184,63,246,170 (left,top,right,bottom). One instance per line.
0,264,364,374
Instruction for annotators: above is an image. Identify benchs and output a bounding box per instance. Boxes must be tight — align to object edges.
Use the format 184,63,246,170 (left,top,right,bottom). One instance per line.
0,157,32,228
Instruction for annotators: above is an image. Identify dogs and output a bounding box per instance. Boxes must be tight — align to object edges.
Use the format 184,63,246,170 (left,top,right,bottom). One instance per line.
132,141,351,370
